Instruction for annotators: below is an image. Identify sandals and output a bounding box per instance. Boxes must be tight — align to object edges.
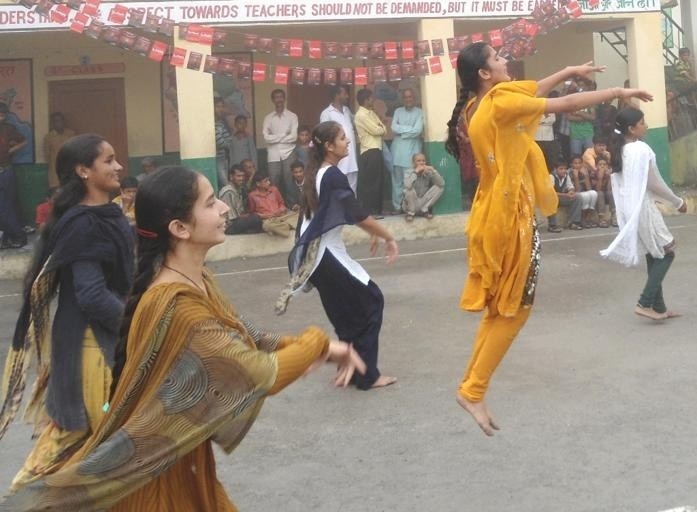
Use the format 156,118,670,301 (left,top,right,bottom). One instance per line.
569,223,582,230
406,214,413,222
548,225,561,232
599,219,608,228
583,221,598,227
0,240,11,250
419,212,433,219
611,218,618,227
11,240,27,247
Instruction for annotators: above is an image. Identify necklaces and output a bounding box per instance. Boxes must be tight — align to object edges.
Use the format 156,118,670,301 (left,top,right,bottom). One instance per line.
323,159,333,166
161,262,204,293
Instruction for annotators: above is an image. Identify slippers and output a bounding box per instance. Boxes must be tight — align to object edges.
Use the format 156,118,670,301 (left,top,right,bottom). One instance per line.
375,215,384,220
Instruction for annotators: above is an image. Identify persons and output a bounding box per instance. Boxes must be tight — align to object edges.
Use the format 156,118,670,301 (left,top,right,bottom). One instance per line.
296,126,309,167
457,106,479,202
672,47,695,93
599,99,617,140
547,161,580,232
388,88,424,215
273,120,398,391
247,170,299,237
535,112,556,174
353,89,387,219
401,153,446,222
286,161,306,210
135,157,158,181
567,153,597,228
262,89,298,188
590,156,617,227
241,158,257,211
582,135,611,175
319,83,360,199
229,115,258,173
548,90,561,161
218,165,262,235
558,80,577,159
34,187,58,242
618,80,631,111
214,96,230,187
444,41,653,437
667,91,674,138
44,112,76,188
111,176,139,227
0,133,138,496
566,78,595,158
0,103,28,249
0,164,368,512
599,106,688,321
